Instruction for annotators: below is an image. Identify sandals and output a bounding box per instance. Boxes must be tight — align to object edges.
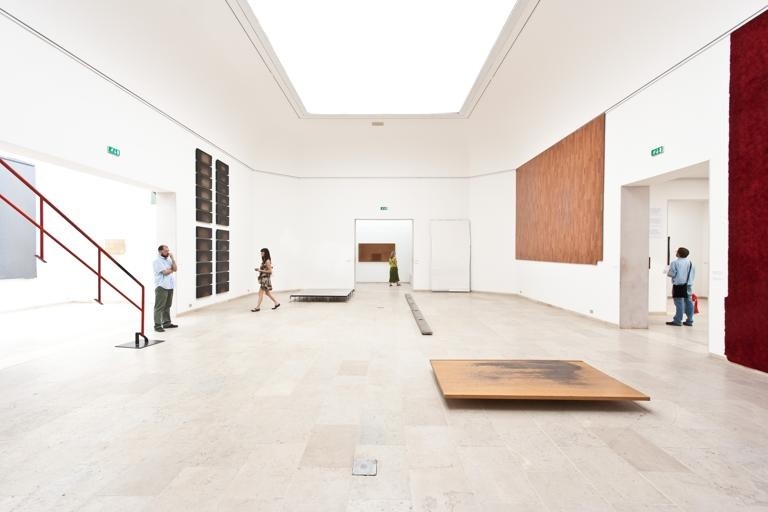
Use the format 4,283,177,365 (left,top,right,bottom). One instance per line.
251,308,261,312
272,303,280,310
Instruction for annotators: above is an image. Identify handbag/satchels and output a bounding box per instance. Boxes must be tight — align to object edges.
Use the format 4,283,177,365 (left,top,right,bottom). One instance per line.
672,283,689,299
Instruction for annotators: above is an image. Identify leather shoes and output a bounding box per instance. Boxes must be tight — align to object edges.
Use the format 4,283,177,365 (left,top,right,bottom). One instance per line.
665,322,693,326
154,325,178,332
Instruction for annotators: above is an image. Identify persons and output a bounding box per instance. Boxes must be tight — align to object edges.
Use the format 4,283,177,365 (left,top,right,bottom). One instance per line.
153,245,178,332
251,248,280,312
388,251,400,286
666,248,695,326
